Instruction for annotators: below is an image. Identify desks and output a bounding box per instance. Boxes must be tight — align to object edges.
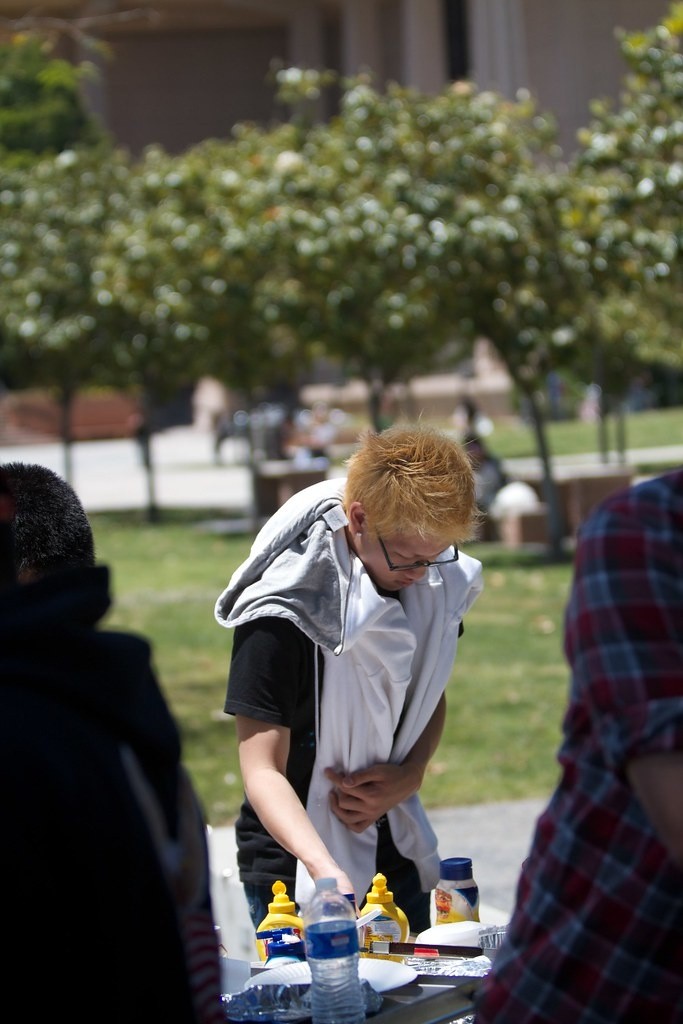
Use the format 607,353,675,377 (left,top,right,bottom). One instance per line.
520,475,636,539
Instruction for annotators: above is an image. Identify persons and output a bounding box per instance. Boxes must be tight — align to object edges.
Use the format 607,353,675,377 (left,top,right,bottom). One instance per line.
472,474,683,1024
462,432,506,542
0,464,230,1024
213,406,484,965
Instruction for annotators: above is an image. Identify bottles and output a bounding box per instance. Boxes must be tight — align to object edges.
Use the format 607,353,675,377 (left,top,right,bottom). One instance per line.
360,873,409,948
435,857,480,927
256,880,304,961
304,878,365,1024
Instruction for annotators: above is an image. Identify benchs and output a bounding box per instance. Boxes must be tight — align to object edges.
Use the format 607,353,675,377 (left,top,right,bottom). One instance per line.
474,515,549,545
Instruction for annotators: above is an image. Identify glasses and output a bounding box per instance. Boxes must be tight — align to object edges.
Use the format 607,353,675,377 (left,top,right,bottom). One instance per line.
373,525,459,572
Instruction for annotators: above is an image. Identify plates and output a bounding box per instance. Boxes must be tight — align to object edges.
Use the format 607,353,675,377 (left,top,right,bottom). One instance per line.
244,958,418,992
